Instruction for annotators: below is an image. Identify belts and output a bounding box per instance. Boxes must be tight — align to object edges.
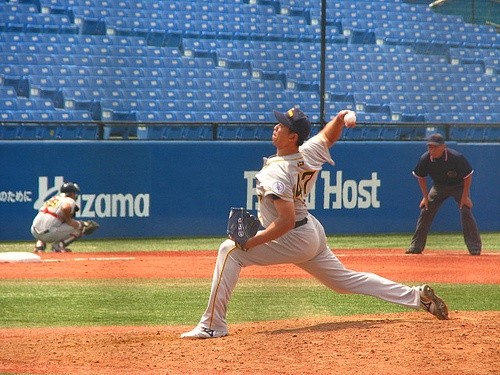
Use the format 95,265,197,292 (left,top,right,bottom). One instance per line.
294,217,308,230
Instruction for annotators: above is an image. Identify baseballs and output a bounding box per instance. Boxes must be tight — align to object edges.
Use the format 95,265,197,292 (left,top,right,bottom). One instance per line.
345,114,357,127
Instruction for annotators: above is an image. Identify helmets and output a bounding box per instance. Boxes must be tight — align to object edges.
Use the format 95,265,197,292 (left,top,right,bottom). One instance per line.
60,182,81,194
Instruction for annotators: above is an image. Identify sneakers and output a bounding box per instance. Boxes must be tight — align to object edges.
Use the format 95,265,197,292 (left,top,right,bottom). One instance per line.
180,325,228,338
420,285,448,320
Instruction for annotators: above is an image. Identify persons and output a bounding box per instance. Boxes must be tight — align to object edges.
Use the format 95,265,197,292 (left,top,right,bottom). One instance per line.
179,106,448,340
31,181,99,253
404,132,482,256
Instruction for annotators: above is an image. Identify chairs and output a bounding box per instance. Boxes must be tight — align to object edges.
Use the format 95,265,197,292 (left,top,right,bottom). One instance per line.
0,0,500,141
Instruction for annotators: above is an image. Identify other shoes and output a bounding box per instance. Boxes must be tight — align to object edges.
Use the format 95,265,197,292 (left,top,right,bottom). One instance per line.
469,250,480,255
35,239,46,253
51,241,72,252
405,247,422,254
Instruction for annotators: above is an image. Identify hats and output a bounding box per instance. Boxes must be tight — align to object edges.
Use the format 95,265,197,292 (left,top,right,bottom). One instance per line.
273,107,311,138
427,134,445,146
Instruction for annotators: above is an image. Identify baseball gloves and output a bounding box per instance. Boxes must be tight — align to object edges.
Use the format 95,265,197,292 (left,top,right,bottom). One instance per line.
227,205,260,244
81,221,100,234
